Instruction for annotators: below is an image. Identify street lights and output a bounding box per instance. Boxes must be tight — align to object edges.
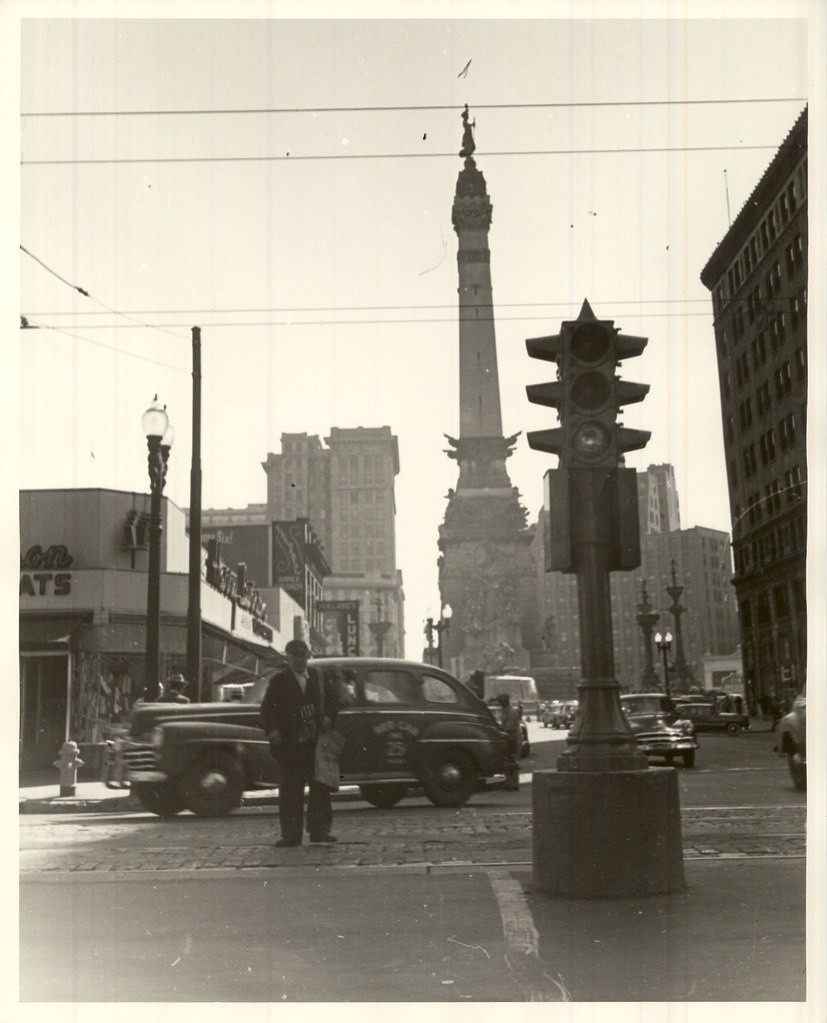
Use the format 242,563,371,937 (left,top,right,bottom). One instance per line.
653,630,674,704
431,603,458,669
139,395,174,700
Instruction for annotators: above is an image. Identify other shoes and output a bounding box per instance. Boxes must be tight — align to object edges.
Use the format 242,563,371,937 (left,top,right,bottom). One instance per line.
276,837,302,847
310,833,338,843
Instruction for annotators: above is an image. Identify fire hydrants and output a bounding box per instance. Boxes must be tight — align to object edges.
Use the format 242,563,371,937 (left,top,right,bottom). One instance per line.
51,738,88,795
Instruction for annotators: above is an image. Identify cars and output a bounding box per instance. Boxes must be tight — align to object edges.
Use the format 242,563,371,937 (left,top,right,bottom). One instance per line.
101,660,515,818
535,693,748,766
771,678,806,791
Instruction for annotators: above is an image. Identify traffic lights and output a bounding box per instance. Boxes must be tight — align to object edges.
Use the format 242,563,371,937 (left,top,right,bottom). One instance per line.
526,319,654,467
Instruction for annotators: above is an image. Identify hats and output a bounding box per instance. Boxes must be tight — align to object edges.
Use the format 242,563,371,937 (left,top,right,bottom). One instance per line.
286,640,308,653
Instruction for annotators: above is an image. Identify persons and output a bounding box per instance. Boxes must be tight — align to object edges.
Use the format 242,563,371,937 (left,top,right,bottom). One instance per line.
495,692,524,790
135,680,164,703
260,640,336,850
157,674,192,703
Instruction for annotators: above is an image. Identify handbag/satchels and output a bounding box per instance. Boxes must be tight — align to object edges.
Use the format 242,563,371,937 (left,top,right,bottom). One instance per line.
316,735,343,793
